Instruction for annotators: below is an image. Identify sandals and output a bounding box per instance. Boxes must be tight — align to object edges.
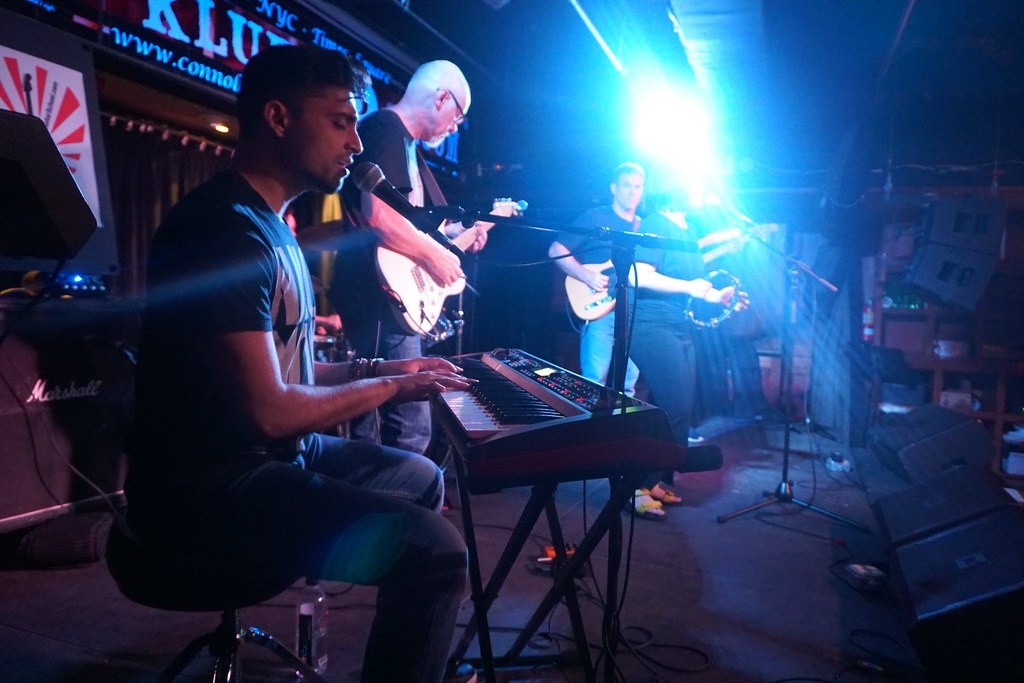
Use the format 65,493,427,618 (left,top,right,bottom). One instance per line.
624,487,665,520
650,483,683,506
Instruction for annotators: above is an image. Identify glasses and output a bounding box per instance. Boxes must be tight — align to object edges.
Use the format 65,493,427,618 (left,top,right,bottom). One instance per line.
437,86,466,125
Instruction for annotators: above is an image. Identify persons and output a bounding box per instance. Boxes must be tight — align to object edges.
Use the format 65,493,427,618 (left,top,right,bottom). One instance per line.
327,56,489,459
118,45,477,683
547,161,646,404
623,170,750,521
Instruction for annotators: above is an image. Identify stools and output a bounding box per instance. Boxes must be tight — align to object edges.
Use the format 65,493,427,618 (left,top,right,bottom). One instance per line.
104,512,328,683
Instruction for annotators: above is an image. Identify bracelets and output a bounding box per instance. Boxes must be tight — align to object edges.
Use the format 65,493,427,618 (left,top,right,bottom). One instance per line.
347,358,386,381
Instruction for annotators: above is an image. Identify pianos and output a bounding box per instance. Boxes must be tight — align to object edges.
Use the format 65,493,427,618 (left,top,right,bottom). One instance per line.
425,342,690,497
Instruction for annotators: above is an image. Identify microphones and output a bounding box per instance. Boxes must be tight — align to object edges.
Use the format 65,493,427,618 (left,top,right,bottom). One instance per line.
351,161,464,255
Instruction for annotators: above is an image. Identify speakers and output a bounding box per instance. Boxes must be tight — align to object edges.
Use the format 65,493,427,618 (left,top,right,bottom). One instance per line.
891,505,1024,683
865,402,998,482
0,296,130,536
865,461,1023,552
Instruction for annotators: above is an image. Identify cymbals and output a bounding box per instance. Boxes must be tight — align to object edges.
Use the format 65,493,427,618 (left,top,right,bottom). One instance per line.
680,268,741,332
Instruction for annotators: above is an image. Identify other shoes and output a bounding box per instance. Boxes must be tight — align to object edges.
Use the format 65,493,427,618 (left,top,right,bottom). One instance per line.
447,664,477,683
442,496,452,510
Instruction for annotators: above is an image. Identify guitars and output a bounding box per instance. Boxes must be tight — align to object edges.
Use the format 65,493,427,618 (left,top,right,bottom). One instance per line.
564,254,619,323
369,195,529,339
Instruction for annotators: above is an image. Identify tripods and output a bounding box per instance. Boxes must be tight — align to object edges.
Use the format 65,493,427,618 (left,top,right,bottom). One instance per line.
697,192,872,532
